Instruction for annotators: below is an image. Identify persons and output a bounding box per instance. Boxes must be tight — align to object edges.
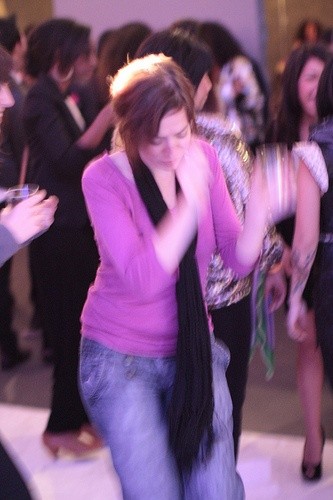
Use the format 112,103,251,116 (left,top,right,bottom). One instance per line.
286,53,333,399
20,17,117,462
0,44,59,499
86,20,155,147
202,22,271,160
0,10,33,372
265,42,327,480
109,29,290,469
76,53,299,499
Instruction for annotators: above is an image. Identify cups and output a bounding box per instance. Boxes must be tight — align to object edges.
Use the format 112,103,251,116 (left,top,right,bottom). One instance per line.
259,143,291,205
6,183,39,207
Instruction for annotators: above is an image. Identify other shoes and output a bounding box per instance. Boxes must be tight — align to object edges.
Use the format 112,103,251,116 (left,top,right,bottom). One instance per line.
301,427,326,481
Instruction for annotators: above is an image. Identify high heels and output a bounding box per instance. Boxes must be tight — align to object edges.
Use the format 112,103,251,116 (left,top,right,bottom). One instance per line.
41,433,99,460
